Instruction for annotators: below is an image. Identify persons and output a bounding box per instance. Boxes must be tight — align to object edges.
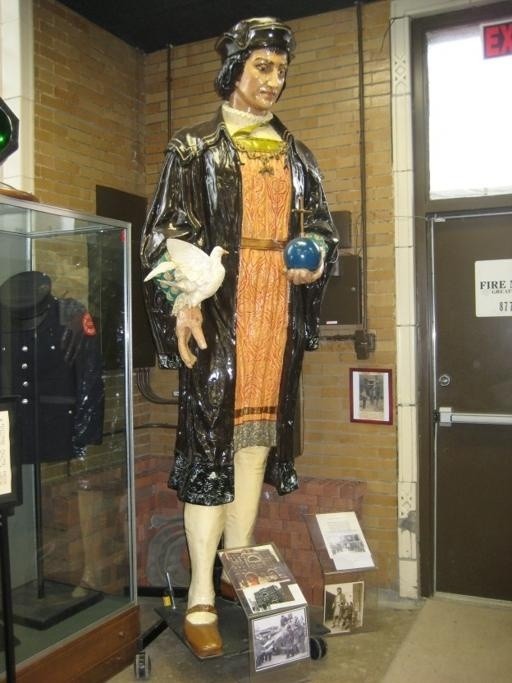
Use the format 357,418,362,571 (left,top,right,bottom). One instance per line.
70,471,106,598
135,12,336,662
248,611,309,670
328,583,357,630
1,269,106,463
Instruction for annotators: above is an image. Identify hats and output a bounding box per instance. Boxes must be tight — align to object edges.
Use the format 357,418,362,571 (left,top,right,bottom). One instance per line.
0,271,52,331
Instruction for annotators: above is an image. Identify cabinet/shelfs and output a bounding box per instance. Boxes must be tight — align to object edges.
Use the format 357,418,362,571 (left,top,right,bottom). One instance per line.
2,188,138,681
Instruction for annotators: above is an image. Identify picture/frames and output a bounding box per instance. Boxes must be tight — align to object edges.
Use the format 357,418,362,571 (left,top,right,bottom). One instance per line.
350,366,393,423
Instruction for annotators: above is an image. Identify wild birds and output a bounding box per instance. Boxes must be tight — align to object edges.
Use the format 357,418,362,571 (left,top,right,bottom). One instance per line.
143,238,230,317
